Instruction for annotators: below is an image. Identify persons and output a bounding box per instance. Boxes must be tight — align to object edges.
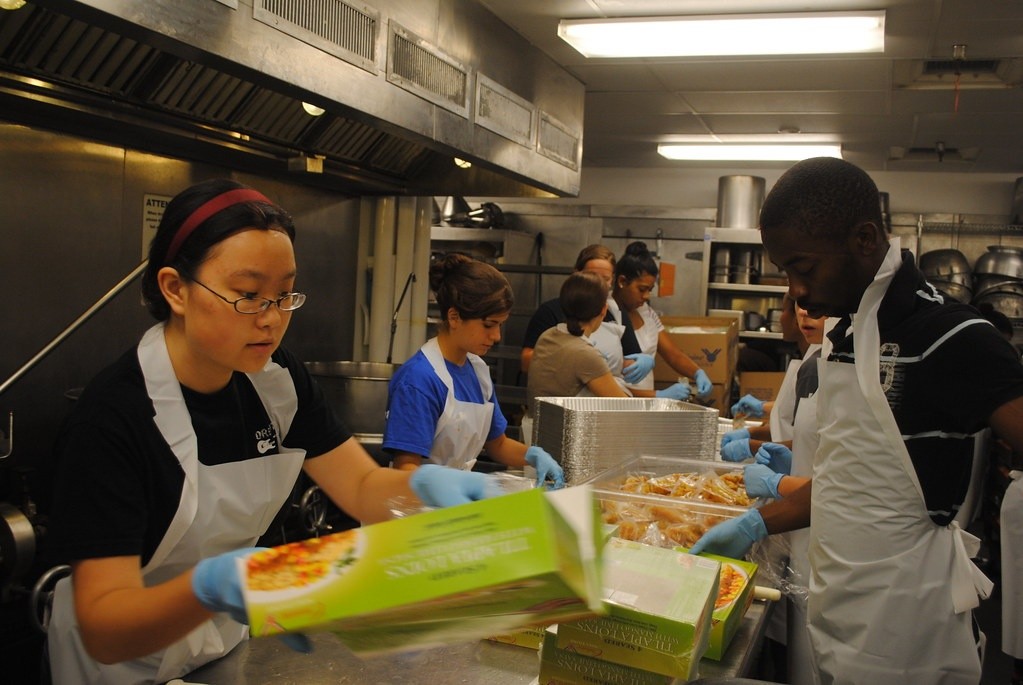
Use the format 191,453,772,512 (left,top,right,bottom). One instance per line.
380,253,564,491
730,292,834,685
685,157,1023,685
39,180,502,685
518,240,714,446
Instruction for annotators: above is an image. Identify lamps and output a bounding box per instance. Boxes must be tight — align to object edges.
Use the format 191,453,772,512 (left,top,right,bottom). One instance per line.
558,8,887,66
658,138,845,170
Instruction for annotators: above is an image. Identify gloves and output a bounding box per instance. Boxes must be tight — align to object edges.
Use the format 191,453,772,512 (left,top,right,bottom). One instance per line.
656,383,692,400
731,394,766,418
689,507,768,560
408,464,506,509
720,438,754,462
743,463,786,499
622,352,655,384
721,428,751,448
525,445,565,490
694,369,713,396
192,547,313,653
755,442,792,476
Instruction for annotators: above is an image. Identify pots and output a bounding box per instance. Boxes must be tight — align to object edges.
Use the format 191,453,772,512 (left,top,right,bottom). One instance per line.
919,245,1023,321
710,250,789,333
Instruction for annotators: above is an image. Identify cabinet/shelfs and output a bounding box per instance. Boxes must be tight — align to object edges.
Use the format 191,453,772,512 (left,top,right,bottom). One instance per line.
427,224,535,429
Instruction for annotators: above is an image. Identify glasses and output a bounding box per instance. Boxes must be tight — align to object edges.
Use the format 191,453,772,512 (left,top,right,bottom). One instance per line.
189,276,306,314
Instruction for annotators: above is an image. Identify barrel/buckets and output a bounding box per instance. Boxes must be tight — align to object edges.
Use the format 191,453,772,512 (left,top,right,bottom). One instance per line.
303,359,402,435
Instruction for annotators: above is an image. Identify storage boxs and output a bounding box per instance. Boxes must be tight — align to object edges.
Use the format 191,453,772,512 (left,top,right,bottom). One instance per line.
660,382,729,417
740,371,789,406
336,486,607,659
242,488,584,636
666,547,759,662
578,453,770,545
551,539,723,678
656,316,740,384
